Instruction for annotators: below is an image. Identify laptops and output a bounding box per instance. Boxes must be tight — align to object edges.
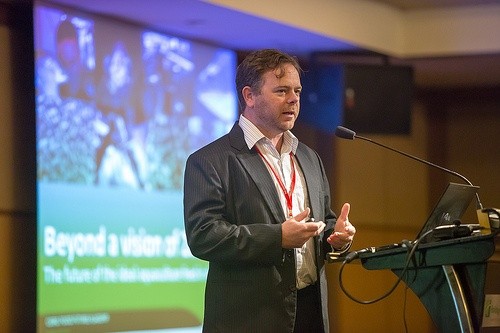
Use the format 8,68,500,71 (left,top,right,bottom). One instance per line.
376,183,480,252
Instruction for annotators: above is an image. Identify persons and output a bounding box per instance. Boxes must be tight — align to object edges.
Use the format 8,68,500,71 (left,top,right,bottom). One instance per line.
183,48,356,333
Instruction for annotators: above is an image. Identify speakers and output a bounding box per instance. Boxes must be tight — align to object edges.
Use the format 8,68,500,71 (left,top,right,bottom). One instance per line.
343,63,413,136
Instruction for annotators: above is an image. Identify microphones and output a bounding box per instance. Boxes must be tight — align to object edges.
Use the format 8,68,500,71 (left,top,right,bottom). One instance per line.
334,125,483,211
